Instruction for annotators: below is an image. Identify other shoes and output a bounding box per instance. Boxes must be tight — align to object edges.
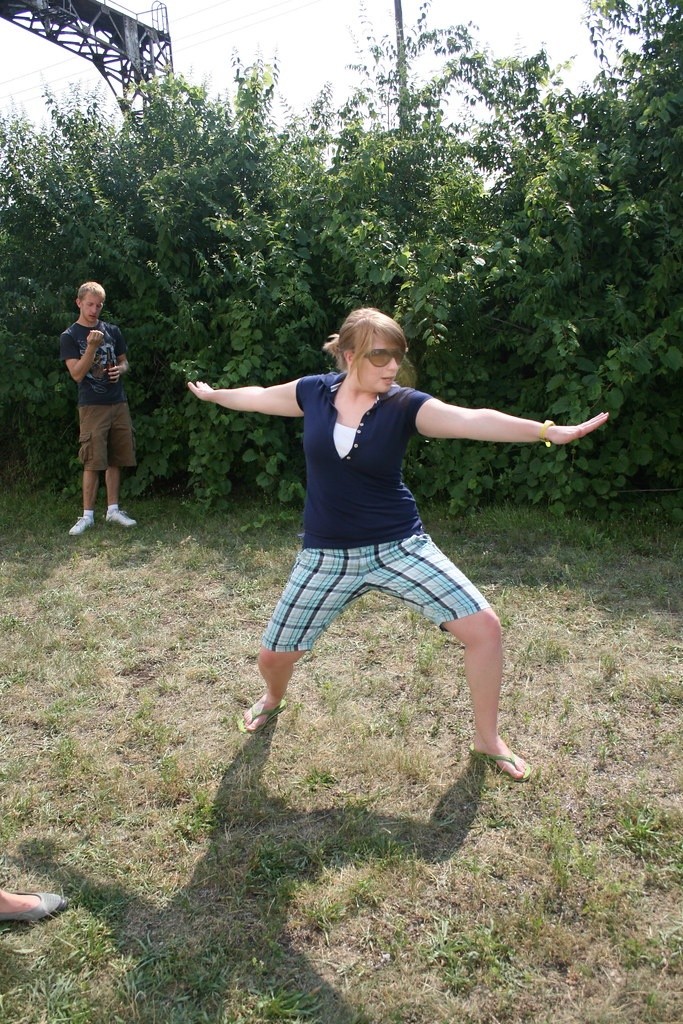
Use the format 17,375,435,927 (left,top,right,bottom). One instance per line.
0,888,62,922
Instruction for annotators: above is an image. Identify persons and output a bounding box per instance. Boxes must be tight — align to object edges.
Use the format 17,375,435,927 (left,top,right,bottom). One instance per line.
0,888,68,923
59,282,138,535
188,309,610,782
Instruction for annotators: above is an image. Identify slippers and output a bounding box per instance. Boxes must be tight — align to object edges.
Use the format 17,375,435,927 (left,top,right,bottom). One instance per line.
469,743,531,782
237,698,288,733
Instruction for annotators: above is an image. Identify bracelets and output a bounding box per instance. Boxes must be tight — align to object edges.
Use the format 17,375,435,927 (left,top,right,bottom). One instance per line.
539,420,556,448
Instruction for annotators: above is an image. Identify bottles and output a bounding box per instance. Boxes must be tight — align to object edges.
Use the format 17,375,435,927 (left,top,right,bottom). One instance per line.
107,347,116,381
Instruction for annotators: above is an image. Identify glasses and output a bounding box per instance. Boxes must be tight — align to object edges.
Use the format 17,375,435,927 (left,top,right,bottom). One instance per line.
360,348,405,367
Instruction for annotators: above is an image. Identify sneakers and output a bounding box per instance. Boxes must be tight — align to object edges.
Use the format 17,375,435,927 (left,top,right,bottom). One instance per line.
106,509,137,527
68,515,94,536
